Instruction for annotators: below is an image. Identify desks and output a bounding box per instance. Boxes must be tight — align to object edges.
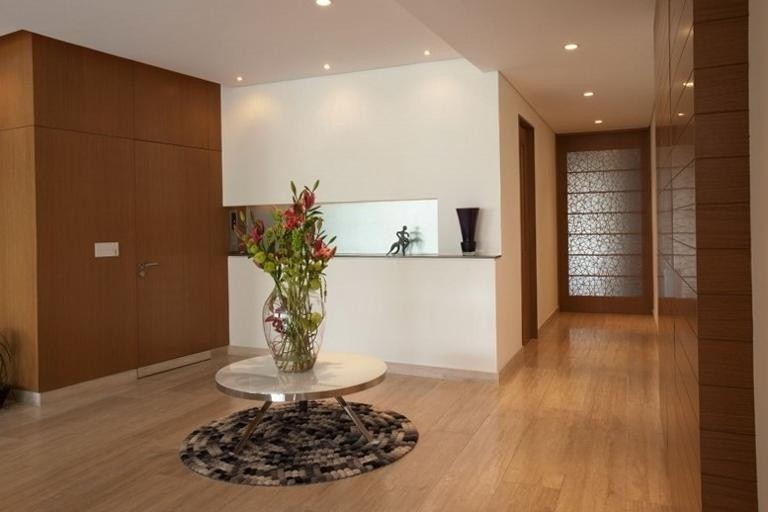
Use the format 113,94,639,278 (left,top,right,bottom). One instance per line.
210,351,388,459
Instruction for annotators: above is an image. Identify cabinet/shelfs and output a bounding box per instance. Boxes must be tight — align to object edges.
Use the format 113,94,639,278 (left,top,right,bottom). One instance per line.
0,27,133,139
0,125,138,405
133,139,230,372
132,58,224,152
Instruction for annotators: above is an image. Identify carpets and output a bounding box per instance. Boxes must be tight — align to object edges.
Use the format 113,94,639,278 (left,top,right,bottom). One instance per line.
177,402,420,489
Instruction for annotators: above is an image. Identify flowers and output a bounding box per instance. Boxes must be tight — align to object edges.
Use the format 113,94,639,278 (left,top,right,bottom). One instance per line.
226,178,340,369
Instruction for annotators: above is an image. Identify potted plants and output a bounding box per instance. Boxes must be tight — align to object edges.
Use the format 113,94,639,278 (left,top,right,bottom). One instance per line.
0,332,20,409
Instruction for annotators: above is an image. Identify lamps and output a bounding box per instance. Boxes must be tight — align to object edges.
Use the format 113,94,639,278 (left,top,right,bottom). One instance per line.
455,205,480,256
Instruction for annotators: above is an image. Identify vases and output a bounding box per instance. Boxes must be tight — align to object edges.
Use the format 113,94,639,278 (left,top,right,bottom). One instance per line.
259,282,327,374
